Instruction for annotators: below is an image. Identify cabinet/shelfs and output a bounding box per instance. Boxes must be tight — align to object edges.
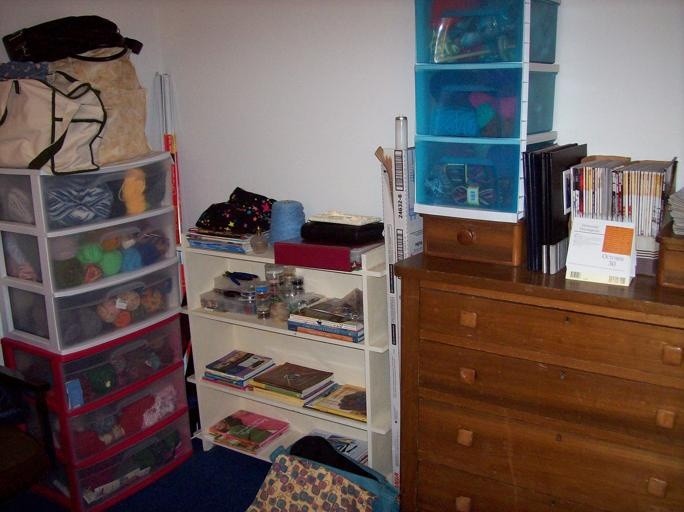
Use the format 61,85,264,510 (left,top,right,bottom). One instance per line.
392,257,684,512
176,239,392,481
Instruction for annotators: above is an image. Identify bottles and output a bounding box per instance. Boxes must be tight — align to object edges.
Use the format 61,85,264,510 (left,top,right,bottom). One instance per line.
252,264,308,320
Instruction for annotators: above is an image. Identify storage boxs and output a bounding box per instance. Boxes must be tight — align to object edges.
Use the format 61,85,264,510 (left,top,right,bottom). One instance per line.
1,152,190,511
414,62,559,136
413,0,557,66
413,130,560,226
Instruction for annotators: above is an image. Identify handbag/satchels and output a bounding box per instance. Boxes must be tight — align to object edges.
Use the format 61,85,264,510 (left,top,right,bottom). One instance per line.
196,186,278,235
271,442,402,512
2,16,117,63
0,46,154,175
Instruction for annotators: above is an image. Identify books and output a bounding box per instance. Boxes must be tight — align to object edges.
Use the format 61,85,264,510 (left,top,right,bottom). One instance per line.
200,274,365,343
520,140,683,288
202,350,367,423
189,222,382,272
208,410,368,464
